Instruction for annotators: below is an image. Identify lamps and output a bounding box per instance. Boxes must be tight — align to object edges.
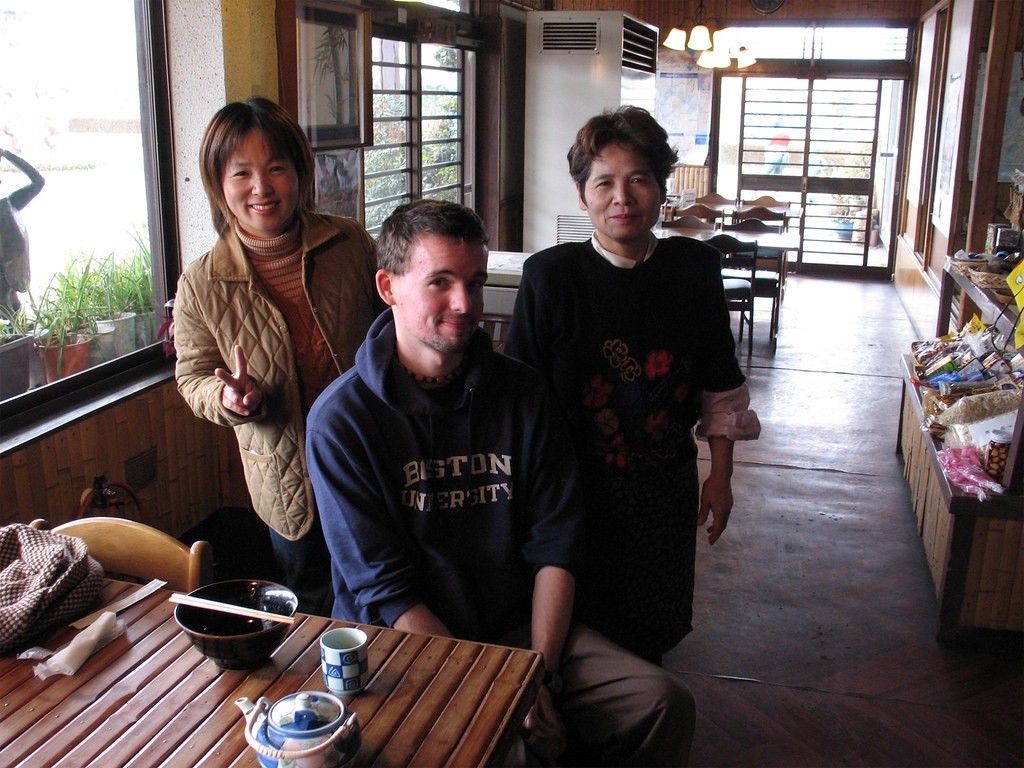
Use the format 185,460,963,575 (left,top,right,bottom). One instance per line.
664,2,758,70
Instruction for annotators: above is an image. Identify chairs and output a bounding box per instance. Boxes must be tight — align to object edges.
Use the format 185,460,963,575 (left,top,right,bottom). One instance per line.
659,192,799,339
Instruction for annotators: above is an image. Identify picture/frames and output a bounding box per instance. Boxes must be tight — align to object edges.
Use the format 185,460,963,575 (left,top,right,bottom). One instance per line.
278,0,377,153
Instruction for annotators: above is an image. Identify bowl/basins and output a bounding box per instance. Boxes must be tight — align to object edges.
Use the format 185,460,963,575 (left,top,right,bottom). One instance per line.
174,579,298,669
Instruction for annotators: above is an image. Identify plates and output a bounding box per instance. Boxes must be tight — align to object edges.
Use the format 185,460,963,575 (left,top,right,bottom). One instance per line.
996,290,1017,306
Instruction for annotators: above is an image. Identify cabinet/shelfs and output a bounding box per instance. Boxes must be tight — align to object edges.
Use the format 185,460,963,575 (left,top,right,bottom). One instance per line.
889,167,1024,646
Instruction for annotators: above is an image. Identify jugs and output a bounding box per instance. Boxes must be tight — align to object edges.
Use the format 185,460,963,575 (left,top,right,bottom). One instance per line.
235,690,362,768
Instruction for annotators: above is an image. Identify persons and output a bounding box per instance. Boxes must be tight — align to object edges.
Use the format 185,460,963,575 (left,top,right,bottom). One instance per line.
304,200,699,768
174,95,390,617
0,146,46,320
505,103,765,673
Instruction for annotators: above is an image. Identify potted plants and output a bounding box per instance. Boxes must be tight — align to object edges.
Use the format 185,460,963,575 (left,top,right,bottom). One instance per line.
0,235,150,404
834,218,854,242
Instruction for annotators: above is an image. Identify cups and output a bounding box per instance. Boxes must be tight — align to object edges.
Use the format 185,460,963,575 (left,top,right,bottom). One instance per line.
319,627,370,695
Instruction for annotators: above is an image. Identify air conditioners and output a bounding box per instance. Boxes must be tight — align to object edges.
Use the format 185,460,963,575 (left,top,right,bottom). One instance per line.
520,6,662,258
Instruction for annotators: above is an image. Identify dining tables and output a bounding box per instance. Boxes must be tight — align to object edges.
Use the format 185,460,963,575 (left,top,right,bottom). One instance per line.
2,548,549,768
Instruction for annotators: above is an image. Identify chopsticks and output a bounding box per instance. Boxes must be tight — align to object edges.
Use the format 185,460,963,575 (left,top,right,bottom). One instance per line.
168,591,295,626
